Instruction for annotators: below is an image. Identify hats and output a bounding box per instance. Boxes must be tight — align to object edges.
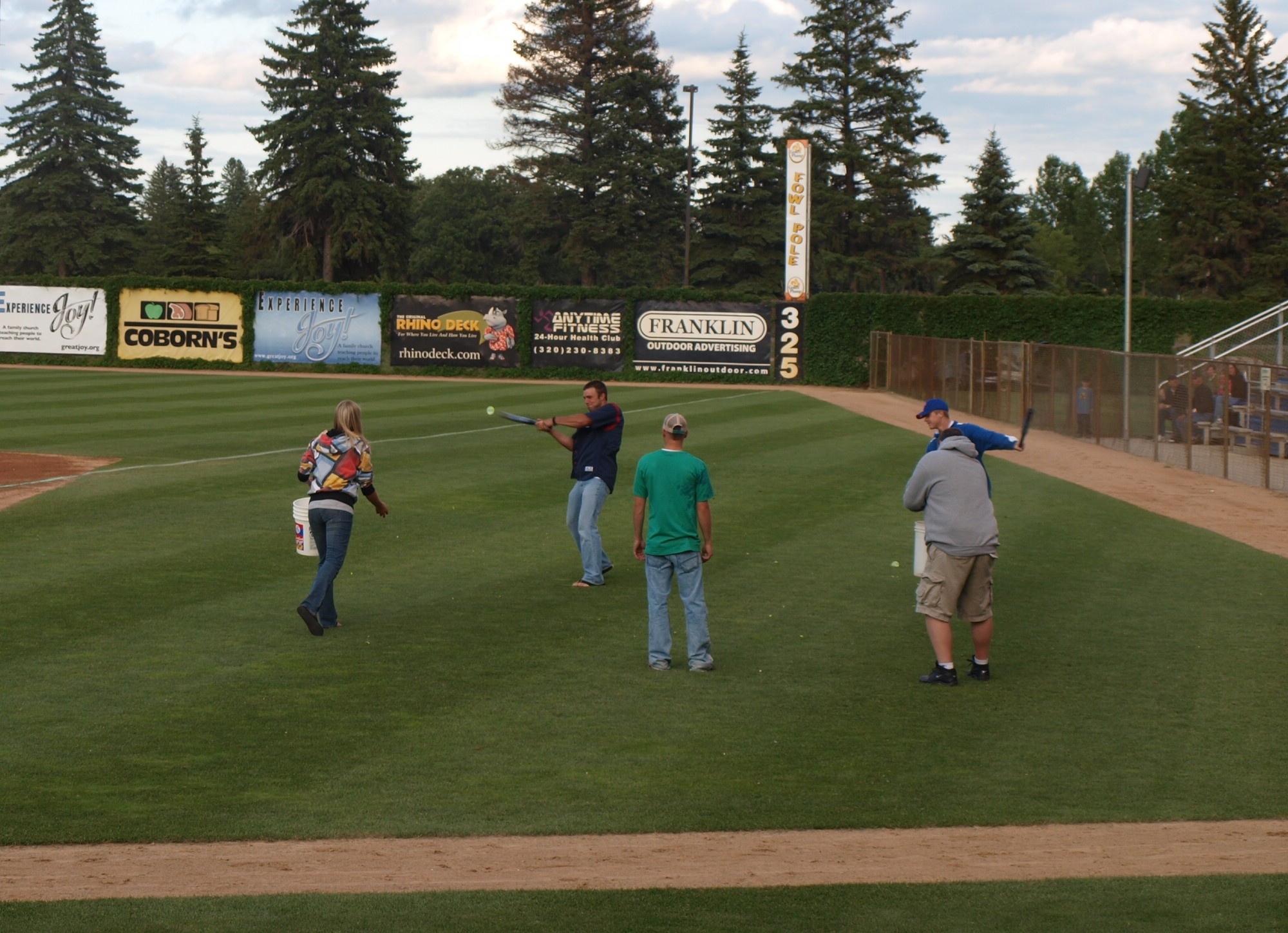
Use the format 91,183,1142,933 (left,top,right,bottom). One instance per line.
662,413,688,434
915,398,949,420
1167,374,1179,380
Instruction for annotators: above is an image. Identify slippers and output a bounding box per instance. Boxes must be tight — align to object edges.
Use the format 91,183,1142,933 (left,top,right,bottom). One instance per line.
602,565,613,574
570,579,593,587
1076,433,1083,438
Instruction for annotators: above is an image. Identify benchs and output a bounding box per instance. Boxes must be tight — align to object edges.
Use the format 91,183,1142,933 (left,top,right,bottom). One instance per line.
1194,385,1288,460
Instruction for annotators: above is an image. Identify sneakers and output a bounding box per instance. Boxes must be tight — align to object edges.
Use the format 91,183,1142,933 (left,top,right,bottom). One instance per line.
966,654,990,681
919,660,959,687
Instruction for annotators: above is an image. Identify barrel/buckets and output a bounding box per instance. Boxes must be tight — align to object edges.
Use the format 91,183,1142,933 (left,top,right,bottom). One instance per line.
292,497,320,556
913,520,928,576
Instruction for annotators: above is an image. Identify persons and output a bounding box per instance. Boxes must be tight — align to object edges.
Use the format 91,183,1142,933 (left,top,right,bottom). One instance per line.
537,380,625,588
296,401,389,635
1206,364,1247,426
633,413,714,670
915,399,1026,498
1077,377,1094,438
1157,376,1188,442
903,428,1000,685
1175,375,1213,441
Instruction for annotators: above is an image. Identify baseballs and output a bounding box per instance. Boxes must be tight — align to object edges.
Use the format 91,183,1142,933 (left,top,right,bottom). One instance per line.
487,406,495,415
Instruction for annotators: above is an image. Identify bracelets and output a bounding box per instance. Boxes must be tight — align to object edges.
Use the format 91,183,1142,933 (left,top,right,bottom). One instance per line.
553,417,557,426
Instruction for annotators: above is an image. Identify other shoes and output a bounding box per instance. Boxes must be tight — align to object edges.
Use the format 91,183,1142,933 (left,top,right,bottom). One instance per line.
1157,434,1167,443
1085,434,1091,439
1190,435,1204,444
1211,421,1223,428
1168,438,1181,443
296,605,324,637
321,622,344,629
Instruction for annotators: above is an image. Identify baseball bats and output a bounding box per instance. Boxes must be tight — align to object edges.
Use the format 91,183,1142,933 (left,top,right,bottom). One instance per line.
1018,408,1034,448
496,410,553,429
